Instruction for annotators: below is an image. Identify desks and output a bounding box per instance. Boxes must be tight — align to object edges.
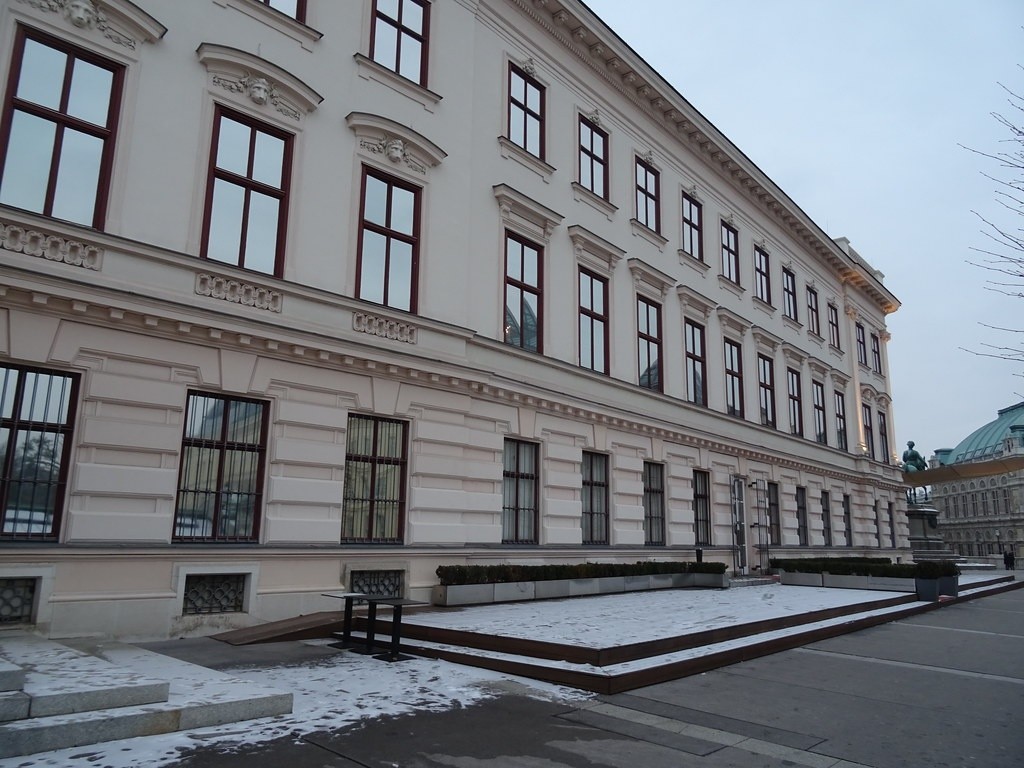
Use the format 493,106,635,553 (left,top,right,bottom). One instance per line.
322,592,430,664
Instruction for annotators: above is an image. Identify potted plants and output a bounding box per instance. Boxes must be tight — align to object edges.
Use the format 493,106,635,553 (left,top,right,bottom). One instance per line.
768,557,963,602
432,561,730,606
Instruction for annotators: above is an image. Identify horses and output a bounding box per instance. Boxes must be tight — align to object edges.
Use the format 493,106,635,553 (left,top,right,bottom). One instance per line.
900,456,928,503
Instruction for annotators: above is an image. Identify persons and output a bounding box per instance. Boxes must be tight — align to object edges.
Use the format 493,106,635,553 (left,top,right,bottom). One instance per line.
902,441,929,472
1003,548,1015,570
